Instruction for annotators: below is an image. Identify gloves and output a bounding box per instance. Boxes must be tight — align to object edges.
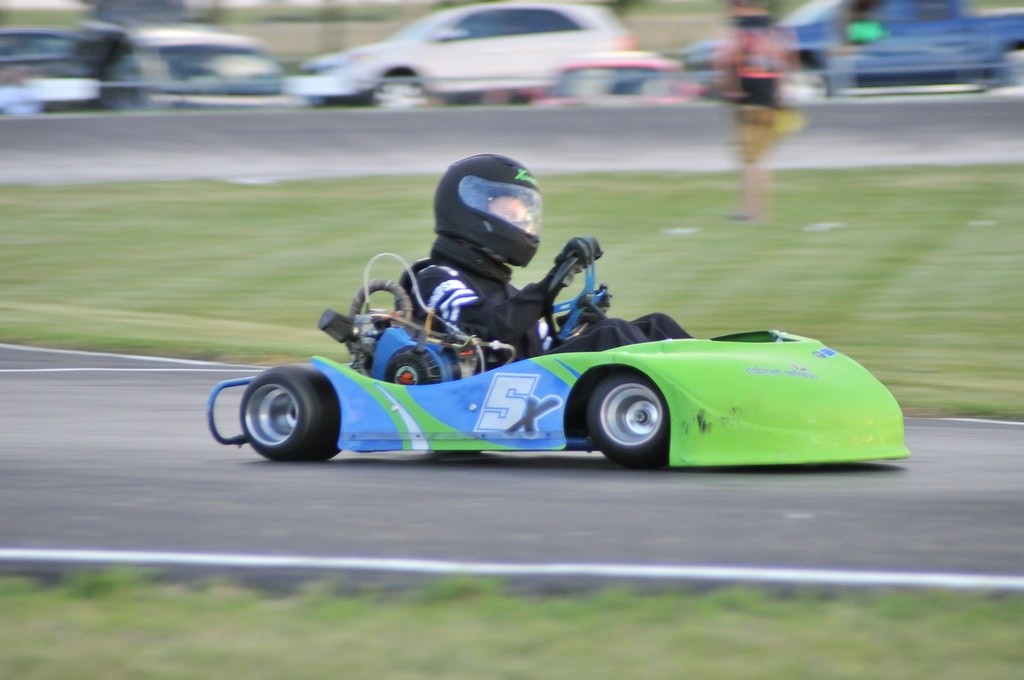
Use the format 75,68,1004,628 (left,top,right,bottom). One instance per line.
555,284,612,328
546,235,604,287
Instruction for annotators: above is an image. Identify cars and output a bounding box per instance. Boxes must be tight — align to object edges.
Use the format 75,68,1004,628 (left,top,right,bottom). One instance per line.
0,2,288,117
534,0,1024,92
284,0,639,107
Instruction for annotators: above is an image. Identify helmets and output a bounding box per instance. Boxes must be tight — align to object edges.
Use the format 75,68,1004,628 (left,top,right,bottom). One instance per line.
434,153,541,268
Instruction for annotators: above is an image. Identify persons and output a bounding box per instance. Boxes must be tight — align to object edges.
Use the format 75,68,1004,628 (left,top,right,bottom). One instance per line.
0,61,45,115
400,153,695,373
712,0,797,222
